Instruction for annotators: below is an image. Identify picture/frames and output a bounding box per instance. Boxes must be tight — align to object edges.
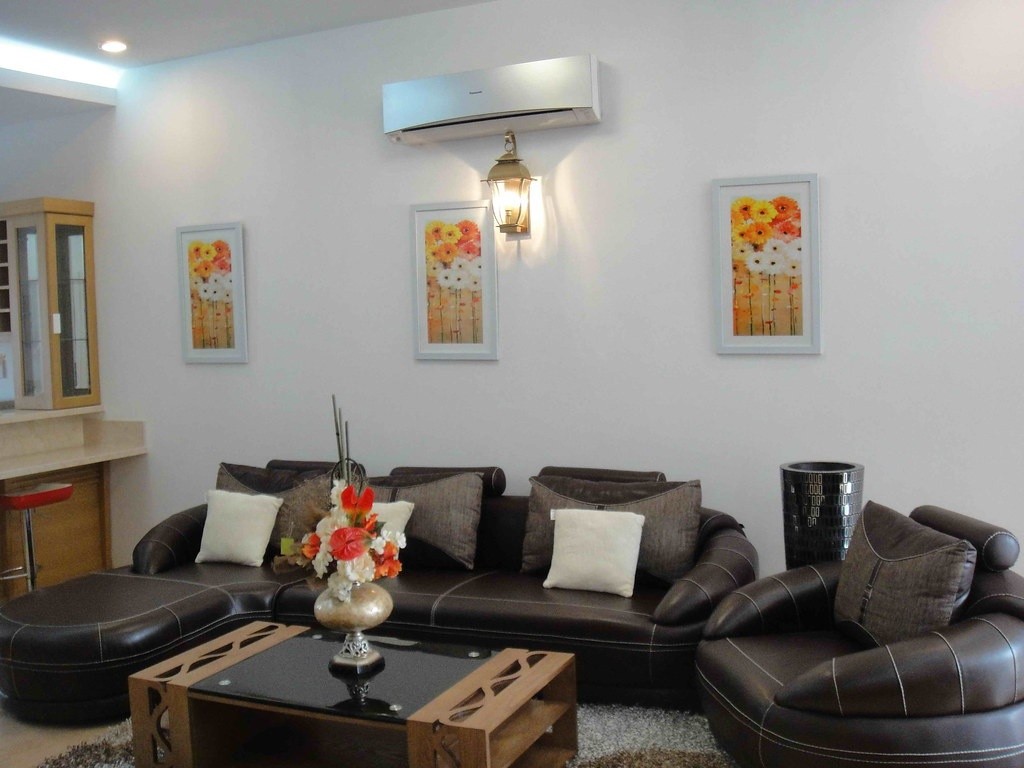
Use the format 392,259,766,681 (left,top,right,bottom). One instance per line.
709,173,819,354
413,200,500,361
176,224,250,365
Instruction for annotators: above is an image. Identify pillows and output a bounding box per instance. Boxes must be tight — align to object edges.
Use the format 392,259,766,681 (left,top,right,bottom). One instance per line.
547,509,646,599
524,474,698,585
194,490,282,566
216,463,332,553
836,500,976,647
361,472,483,571
302,499,413,564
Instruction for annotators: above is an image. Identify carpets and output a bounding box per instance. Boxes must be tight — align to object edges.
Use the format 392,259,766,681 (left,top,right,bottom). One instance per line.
39,703,743,768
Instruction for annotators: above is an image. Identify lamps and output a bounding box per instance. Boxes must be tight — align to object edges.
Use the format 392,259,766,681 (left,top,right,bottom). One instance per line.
482,130,535,234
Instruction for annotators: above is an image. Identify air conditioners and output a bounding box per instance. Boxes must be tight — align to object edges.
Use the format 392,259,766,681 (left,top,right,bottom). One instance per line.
381,55,599,144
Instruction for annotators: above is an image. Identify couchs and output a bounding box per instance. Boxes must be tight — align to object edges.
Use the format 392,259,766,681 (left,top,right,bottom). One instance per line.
0,465,762,727
696,504,1024,768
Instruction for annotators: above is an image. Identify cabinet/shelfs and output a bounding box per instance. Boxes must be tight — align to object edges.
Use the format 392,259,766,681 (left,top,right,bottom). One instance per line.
1,459,117,609
0,200,100,410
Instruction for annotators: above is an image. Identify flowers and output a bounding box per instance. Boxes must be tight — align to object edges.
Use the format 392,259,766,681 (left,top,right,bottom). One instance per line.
286,478,403,603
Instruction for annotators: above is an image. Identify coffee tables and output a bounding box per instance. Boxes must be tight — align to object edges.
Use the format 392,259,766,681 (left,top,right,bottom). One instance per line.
127,622,580,768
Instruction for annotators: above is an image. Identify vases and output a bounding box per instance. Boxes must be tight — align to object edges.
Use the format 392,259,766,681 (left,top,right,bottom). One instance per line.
779,461,865,573
314,580,393,705
